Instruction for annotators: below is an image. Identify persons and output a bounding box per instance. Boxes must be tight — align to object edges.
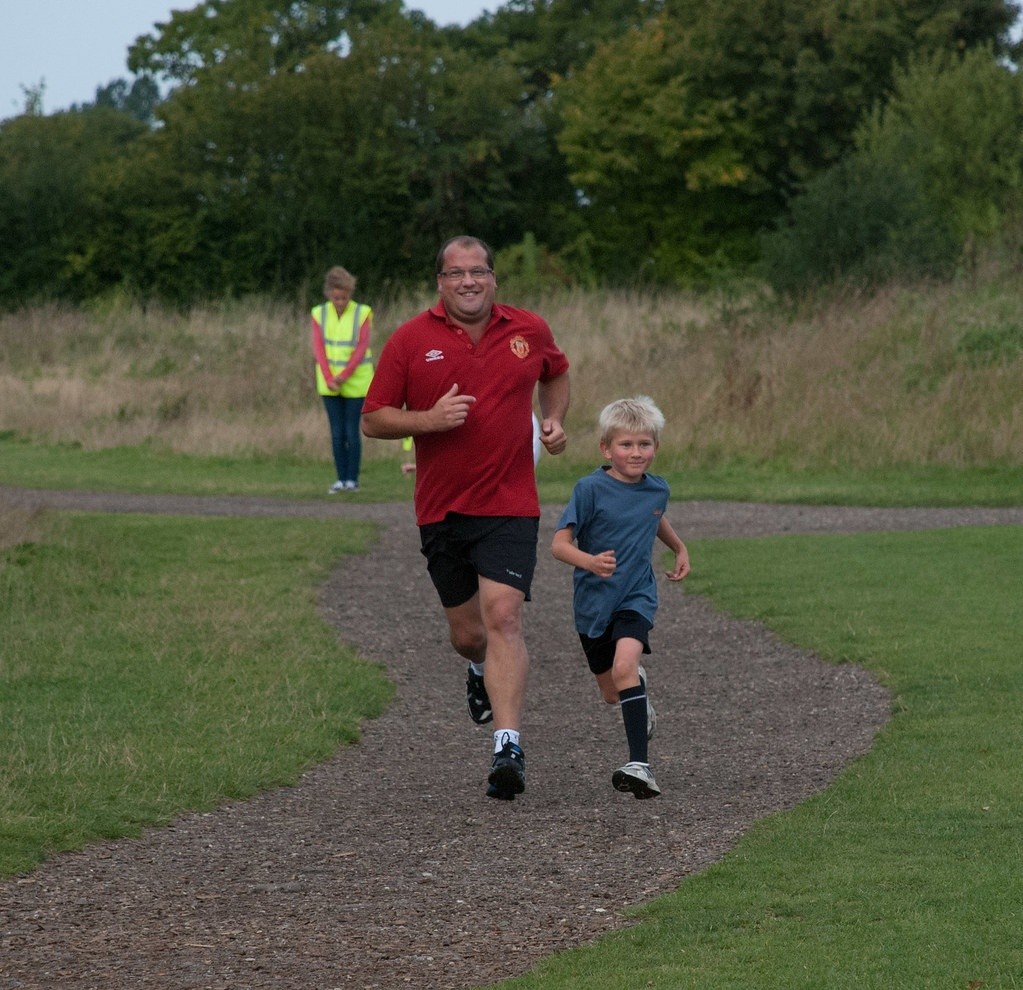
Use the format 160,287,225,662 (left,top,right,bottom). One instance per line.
358,236,570,801
549,396,692,801
309,265,375,495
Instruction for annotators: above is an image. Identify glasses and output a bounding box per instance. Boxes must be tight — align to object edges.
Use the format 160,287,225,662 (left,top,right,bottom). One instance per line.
441,268,493,281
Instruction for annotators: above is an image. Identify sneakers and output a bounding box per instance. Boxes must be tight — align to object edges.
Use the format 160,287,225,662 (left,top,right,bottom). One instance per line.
638,665,657,740
465,661,493,725
343,480,360,492
485,742,526,802
329,480,344,495
611,761,661,799
